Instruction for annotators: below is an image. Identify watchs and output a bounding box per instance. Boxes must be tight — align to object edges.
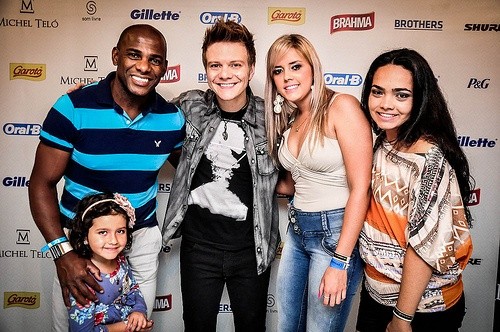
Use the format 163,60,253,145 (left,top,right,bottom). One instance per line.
124,318,129,327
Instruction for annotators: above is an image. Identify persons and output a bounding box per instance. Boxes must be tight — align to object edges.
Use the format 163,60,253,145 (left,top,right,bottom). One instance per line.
67,191,154,332
29,24,187,332
265,34,373,332
353,49,476,332
67,18,289,332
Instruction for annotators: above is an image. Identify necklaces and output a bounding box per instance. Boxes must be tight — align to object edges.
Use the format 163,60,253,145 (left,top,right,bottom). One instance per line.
217,101,249,141
293,112,311,131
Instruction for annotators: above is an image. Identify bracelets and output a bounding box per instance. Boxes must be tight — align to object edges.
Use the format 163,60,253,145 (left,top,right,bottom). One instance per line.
393,305,414,322
330,251,350,270
41,237,73,261
